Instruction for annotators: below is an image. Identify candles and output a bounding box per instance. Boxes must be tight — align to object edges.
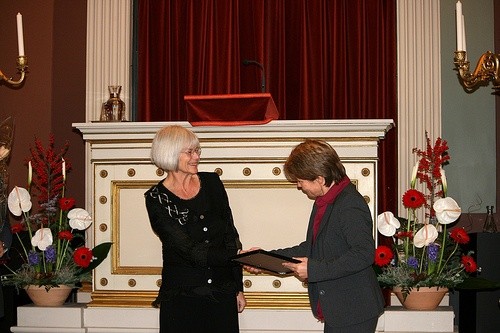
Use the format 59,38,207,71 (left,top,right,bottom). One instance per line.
16,11,25,56
462,15,468,61
455,0,463,51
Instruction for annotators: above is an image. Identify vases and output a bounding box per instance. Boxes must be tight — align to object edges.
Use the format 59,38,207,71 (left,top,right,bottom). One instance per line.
483,205,497,232
21,285,73,308
392,286,449,311
102,86,126,121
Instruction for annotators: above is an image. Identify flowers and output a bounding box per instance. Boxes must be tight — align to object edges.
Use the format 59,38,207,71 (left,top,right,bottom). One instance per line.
0,135,114,293
373,128,483,298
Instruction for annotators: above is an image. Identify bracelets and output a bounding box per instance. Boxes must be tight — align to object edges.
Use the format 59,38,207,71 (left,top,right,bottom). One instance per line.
237,249,241,255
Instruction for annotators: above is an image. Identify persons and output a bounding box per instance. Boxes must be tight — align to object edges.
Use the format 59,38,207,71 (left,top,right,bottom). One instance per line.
144,125,262,333
251,140,384,333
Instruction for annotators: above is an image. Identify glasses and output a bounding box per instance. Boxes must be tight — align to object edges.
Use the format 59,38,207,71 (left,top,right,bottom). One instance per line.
182,147,201,155
